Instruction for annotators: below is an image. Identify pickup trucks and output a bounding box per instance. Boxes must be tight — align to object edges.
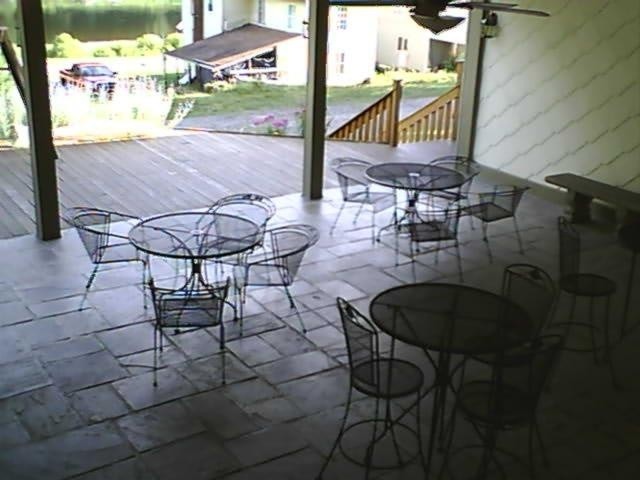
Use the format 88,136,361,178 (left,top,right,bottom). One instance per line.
59,62,119,95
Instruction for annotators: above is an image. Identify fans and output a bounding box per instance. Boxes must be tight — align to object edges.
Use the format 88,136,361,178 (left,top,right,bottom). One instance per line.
329,0,551,18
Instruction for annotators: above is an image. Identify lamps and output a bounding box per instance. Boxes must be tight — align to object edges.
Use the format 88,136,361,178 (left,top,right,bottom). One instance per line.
480,13,499,39
409,14,466,34
